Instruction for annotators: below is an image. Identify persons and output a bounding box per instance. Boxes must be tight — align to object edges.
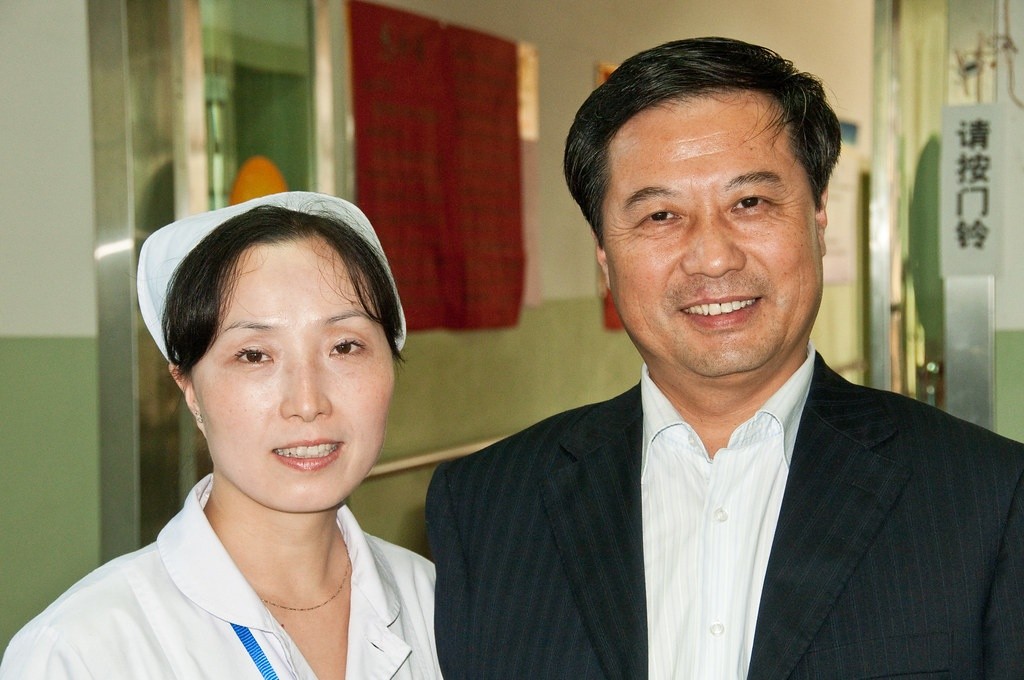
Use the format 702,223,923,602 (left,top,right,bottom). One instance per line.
0,190,445,680
422,35,1024,680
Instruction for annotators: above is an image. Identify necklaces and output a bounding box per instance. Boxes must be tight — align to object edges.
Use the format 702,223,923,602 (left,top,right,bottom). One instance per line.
264,558,352,612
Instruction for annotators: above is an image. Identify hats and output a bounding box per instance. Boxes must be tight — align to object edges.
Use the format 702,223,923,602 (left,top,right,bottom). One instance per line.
138,192,408,361
